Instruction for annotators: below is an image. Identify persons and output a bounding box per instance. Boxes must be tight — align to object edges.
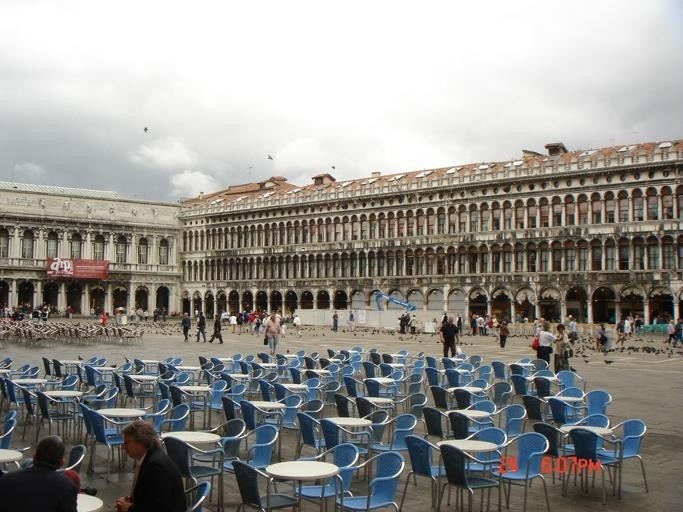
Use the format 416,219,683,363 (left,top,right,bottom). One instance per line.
0,435,77,511
0,300,683,374
114,421,188,512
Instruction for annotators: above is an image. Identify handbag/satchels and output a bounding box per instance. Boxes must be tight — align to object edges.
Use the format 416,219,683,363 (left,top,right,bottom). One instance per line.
531,337,539,349
560,343,573,357
264,335,268,345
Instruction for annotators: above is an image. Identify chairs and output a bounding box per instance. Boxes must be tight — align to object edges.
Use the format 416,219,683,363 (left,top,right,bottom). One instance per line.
1,317,146,350
